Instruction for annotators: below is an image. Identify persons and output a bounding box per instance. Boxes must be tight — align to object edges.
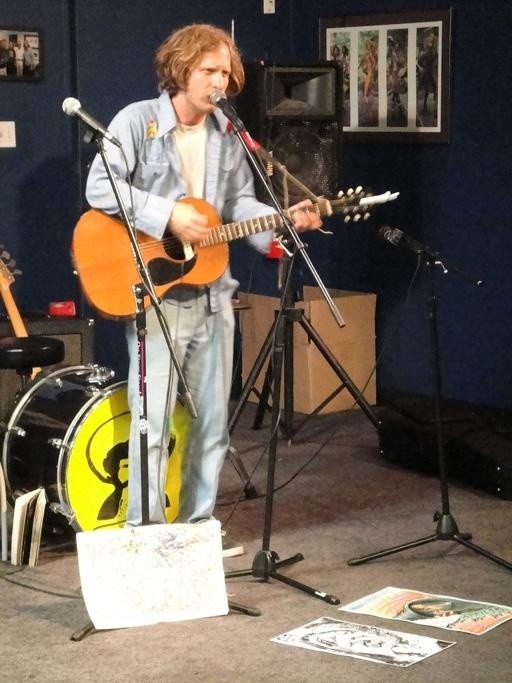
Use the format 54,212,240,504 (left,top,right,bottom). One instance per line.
85,20,323,560
0,33,36,77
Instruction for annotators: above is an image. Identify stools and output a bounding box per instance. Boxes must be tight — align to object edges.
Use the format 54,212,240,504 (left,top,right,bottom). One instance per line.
0,337,65,390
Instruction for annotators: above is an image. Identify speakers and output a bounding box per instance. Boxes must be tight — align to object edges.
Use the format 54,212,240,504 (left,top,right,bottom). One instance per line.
0,315,97,429
240,55,344,213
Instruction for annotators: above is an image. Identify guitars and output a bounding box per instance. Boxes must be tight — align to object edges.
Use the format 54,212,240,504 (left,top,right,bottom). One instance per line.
72,184,401,323
0,245,43,383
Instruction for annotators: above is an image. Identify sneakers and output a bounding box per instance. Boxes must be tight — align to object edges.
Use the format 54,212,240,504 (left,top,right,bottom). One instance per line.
221,530,244,558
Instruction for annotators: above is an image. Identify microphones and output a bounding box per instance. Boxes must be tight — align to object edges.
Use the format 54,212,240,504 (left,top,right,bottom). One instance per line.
61,95,126,146
206,90,245,132
380,220,439,263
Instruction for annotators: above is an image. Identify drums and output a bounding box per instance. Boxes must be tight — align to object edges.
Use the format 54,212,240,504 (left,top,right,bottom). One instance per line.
2,363,192,538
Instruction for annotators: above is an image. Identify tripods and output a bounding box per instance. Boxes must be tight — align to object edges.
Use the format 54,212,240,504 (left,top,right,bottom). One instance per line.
347,265,512,586
223,231,383,463
66,134,263,643
217,129,341,608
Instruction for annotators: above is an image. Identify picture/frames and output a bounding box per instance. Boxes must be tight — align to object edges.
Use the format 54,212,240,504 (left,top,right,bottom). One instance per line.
0,26,45,82
318,8,454,146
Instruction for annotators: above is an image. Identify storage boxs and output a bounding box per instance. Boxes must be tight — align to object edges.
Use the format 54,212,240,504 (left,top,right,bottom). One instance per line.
237,285,377,415
